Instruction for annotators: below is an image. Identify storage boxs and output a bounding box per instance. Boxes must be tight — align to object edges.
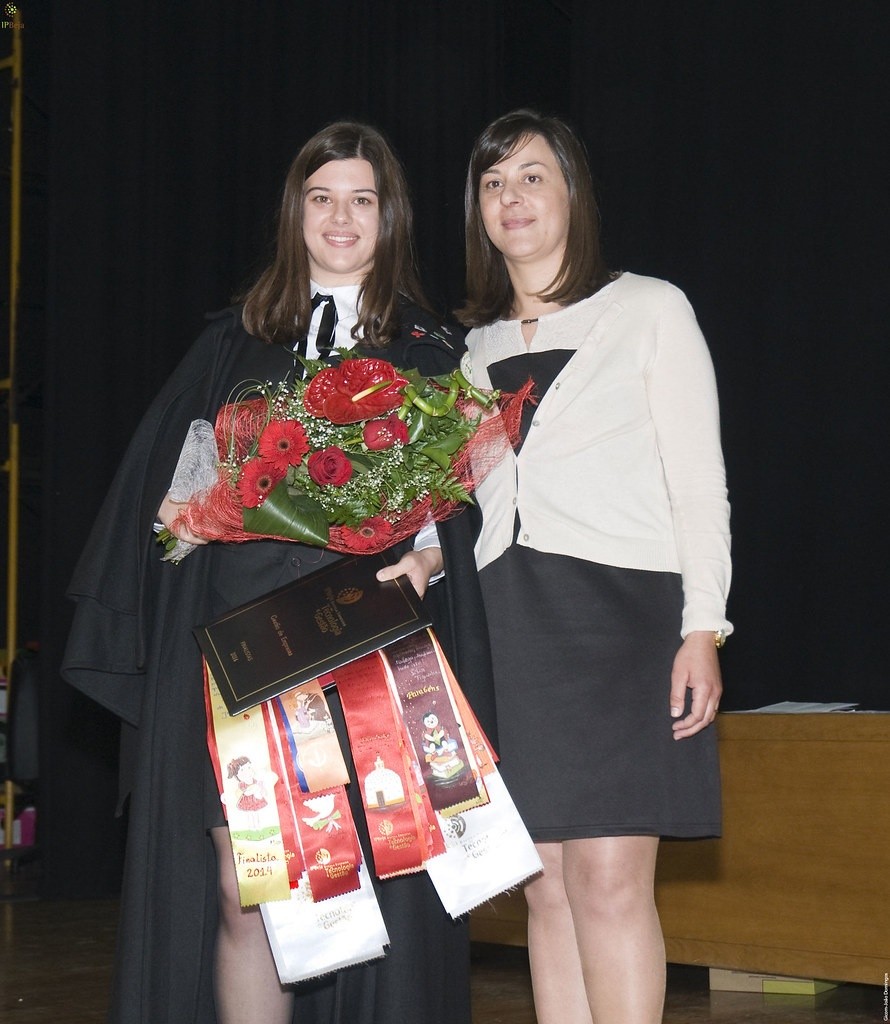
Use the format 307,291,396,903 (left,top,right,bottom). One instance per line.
710,967,842,996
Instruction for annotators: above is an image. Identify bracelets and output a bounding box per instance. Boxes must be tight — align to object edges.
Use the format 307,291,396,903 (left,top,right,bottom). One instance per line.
714,629,727,648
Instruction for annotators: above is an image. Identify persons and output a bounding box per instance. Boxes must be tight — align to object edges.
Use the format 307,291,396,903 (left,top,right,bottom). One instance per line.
66,121,486,1024
459,110,735,1024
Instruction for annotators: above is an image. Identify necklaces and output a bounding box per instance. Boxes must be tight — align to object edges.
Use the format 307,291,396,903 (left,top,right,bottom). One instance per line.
509,300,539,322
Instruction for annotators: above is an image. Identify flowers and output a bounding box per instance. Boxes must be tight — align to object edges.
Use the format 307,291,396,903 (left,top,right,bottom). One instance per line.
156,345,501,564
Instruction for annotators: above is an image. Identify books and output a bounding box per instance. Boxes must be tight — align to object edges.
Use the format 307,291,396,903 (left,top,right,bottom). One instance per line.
193,544,435,717
